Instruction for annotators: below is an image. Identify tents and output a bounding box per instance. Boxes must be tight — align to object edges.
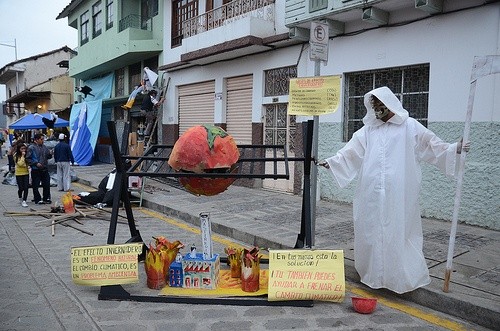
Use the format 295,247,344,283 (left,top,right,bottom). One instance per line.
8,113,70,130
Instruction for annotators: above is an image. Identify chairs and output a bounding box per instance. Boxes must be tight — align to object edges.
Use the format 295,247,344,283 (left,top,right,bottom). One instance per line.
122,176,146,210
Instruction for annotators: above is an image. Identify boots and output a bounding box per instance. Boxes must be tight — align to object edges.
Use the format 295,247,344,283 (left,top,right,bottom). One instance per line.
124,99,135,108
121,102,129,110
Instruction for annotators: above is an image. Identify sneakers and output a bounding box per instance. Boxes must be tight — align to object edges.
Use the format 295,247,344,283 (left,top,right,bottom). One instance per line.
21,200,28,208
19,197,24,204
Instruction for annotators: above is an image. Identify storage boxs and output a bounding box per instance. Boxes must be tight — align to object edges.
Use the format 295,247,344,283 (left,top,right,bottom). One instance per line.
182,253,220,289
169,260,183,287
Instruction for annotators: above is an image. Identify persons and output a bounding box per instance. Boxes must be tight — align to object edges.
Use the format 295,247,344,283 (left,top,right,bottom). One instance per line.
13,143,29,207
1,128,73,158
24,133,52,204
121,67,153,110
73,86,86,104
54,133,74,192
33,110,60,140
315,87,471,294
141,90,166,136
0,136,26,175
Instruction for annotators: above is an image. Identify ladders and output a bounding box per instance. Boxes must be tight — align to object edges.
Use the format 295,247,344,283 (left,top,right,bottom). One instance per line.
142,77,170,151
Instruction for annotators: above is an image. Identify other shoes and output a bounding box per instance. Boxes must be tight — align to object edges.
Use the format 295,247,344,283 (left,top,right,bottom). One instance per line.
37,200,44,204
43,199,52,204
